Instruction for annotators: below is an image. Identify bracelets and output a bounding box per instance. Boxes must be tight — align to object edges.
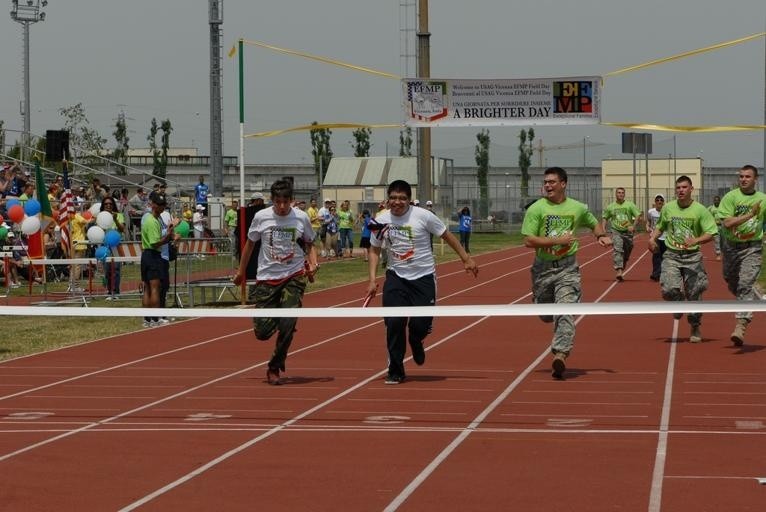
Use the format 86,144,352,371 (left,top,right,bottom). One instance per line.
597,234,605,240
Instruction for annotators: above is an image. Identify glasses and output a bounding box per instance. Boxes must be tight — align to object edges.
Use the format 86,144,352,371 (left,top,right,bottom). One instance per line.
104,203,112,205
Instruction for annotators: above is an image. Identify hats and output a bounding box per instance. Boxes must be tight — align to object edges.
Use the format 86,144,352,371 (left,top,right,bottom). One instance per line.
251,192,266,200
153,193,169,206
196,204,206,210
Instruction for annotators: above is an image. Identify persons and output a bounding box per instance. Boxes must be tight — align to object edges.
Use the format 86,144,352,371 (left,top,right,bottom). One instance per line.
649,176,721,343
603,187,642,281
645,195,668,281
520,167,614,377
225,178,353,304
233,178,321,384
714,165,766,347
2,158,211,329
359,179,479,384
708,195,723,260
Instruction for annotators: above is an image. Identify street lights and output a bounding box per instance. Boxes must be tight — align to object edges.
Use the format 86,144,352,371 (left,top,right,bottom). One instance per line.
504,171,510,210
506,183,512,222
9,0,47,164
196,112,202,158
583,135,590,206
696,150,705,158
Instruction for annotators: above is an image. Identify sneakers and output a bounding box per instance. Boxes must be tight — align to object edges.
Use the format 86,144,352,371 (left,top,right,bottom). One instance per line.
267,368,283,385
384,367,405,385
144,318,170,327
410,342,425,364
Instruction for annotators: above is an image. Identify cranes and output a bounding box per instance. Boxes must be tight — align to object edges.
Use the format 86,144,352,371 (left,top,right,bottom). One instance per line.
521,135,605,167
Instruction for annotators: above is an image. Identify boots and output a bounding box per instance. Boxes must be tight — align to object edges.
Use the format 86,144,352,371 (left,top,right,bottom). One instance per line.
616,269,624,282
551,353,567,379
689,323,702,343
730,318,748,346
